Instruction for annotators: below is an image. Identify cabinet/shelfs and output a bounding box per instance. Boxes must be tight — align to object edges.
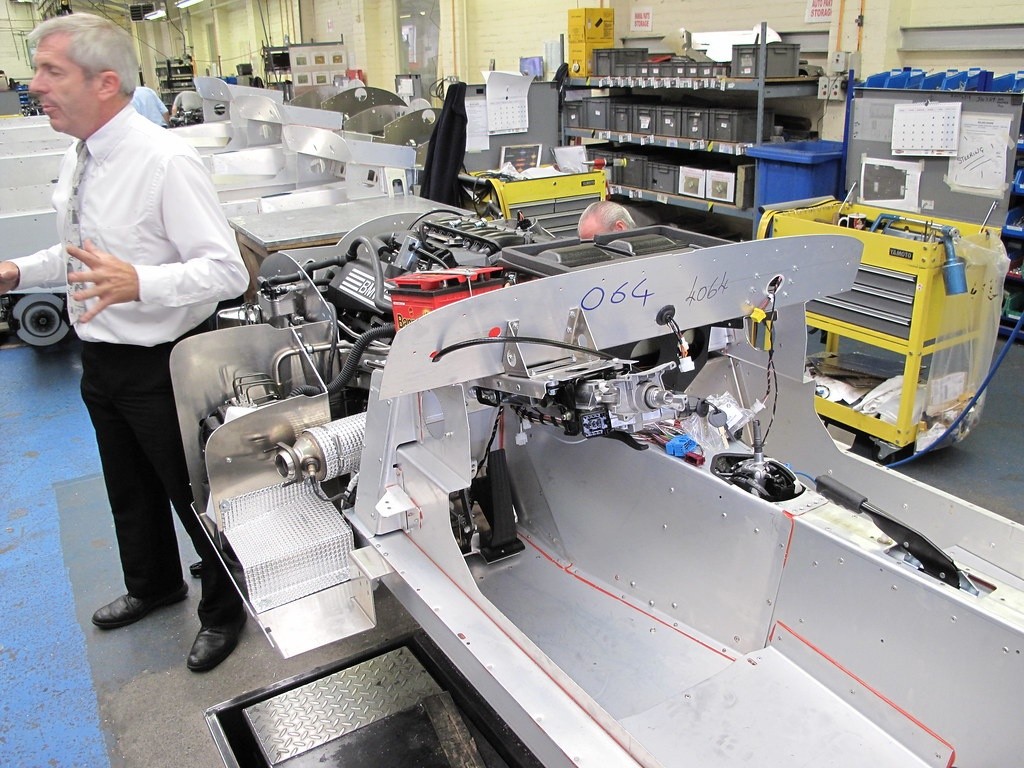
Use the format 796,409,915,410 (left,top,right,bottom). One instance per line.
560,22,822,241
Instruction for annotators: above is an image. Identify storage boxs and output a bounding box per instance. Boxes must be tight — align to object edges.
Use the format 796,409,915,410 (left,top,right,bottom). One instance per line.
565,7,803,202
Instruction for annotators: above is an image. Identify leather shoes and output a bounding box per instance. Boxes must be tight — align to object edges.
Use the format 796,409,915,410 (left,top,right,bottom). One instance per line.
92,578,189,628
186,625,240,668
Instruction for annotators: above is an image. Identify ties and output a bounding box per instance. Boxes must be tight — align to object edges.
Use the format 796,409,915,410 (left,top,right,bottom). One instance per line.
65,141,89,324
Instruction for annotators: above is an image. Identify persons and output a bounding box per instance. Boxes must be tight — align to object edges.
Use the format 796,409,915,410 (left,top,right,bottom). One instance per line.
172,91,203,118
578,201,637,240
131,87,169,129
0,12,250,673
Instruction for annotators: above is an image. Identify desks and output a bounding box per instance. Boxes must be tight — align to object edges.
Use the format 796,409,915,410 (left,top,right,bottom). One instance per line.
228,194,477,300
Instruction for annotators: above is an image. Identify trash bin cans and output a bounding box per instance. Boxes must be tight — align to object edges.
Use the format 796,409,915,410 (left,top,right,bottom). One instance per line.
747,140,843,240
